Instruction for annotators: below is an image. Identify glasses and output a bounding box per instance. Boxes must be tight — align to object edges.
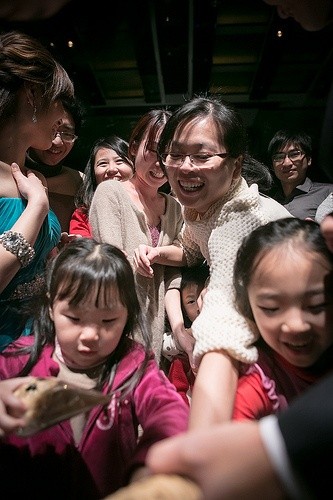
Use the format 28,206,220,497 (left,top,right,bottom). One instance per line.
271,151,305,162
54,130,77,142
159,151,230,167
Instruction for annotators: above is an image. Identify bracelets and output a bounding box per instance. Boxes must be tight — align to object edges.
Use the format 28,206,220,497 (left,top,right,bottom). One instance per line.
0,230,36,267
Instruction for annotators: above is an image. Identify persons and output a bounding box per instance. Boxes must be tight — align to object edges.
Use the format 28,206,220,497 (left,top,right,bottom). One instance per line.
162,270,217,411
253,129,332,219
106,95,298,499
313,189,333,257
18,93,89,242
223,216,333,425
0,31,75,366
69,132,136,240
145,362,333,500
86,109,187,379
0,238,193,500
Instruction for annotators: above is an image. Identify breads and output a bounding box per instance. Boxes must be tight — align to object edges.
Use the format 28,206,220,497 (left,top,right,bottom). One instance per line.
6,376,112,436
